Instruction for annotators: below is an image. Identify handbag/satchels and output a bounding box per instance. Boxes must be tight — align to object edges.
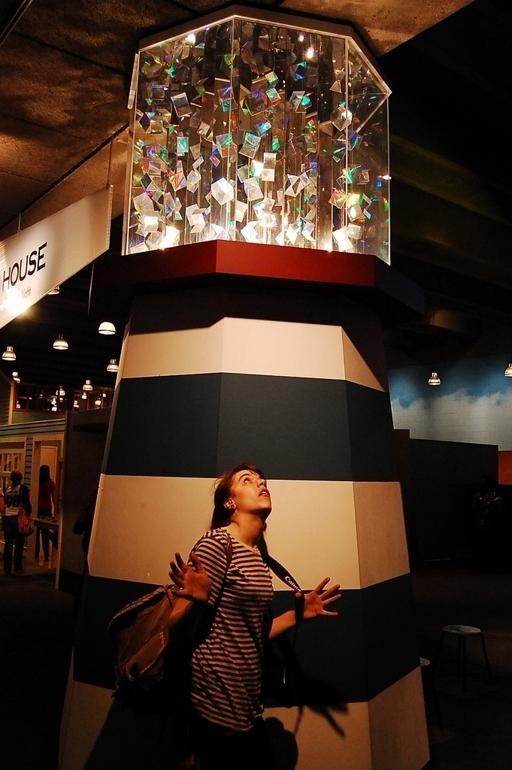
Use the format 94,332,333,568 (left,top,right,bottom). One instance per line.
18,509,35,536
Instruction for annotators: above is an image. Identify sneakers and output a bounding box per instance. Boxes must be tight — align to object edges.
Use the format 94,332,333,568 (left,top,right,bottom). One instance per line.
4,567,24,577
35,553,50,561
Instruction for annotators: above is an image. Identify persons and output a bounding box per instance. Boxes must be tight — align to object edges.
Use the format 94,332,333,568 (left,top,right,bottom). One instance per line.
3,469,33,577
155,461,342,770
36,464,57,563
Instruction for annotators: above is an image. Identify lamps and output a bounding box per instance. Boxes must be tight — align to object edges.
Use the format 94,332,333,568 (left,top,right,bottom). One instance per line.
2,343,18,362
503,362,512,377
427,371,441,387
53,332,70,352
56,357,121,396
99,320,118,336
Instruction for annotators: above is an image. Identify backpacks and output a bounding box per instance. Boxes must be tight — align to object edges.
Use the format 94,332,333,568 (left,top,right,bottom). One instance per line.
102,526,234,695
